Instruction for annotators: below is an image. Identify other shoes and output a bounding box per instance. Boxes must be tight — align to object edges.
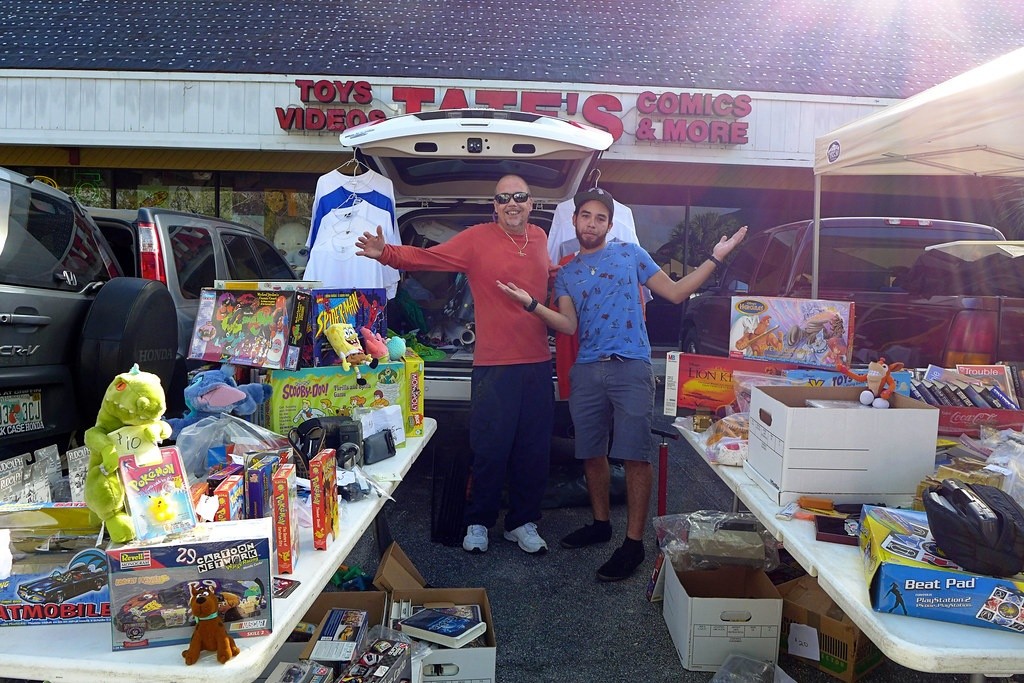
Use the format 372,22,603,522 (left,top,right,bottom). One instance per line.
559,526,612,548
596,546,645,581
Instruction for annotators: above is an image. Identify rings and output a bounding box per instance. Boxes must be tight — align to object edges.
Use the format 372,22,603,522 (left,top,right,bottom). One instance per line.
363,244,366,249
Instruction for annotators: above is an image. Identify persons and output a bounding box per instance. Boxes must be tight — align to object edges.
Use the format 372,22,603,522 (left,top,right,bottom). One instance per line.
496,188,748,581
356,175,564,554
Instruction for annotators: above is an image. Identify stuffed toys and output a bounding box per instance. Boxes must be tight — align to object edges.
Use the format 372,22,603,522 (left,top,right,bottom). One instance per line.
836,358,904,409
181,585,239,666
167,365,273,476
324,323,379,386
360,326,390,364
385,336,406,361
83,363,173,543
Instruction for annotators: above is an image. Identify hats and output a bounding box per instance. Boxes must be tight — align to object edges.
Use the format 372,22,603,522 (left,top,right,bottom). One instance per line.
574,187,614,218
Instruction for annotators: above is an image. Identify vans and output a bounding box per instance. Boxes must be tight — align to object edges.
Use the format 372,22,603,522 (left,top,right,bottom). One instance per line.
83,205,301,386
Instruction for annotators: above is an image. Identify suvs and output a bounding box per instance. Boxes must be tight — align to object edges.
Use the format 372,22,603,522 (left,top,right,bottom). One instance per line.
335,107,614,429
0,166,179,466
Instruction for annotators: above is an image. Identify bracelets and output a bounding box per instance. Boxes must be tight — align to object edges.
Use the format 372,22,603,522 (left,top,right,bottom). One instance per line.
708,255,721,266
523,297,538,312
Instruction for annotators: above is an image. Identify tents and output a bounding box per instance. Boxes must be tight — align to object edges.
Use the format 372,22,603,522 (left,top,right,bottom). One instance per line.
815,44,1024,351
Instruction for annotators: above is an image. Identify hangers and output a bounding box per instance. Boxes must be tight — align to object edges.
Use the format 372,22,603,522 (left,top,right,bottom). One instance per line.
590,168,601,192
333,147,371,240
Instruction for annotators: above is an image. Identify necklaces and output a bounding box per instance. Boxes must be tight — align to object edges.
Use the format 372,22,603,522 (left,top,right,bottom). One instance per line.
504,228,529,257
579,250,607,276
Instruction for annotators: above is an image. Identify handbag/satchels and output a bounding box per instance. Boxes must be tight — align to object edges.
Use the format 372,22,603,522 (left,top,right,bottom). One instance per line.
922,483,1024,578
288,426,327,479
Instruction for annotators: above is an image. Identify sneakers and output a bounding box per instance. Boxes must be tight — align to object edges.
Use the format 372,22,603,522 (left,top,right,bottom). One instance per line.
463,524,488,552
504,521,549,553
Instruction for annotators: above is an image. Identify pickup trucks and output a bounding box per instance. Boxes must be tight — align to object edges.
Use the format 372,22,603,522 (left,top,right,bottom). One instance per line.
678,216,1023,370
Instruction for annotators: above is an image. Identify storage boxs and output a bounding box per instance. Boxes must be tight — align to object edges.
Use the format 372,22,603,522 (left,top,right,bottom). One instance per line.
0,278,496,683
653,295,1024,683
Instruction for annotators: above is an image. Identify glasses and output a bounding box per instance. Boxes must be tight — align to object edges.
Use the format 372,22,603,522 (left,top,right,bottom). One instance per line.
494,192,530,204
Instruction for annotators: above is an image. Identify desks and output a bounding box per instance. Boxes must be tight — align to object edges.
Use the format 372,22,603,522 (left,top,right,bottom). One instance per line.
0,417,437,683
673,411,1024,683
